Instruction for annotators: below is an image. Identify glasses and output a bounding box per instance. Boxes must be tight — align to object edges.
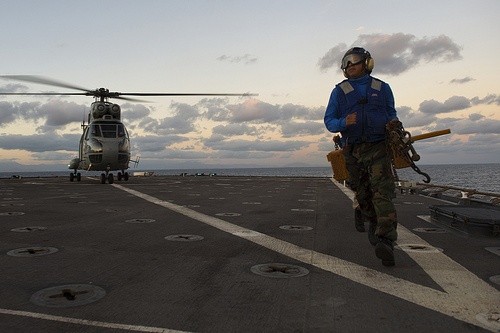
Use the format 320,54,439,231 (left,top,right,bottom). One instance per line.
343,53,366,69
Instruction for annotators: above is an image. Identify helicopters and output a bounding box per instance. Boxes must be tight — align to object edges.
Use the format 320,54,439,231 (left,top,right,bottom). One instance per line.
0,74,260,185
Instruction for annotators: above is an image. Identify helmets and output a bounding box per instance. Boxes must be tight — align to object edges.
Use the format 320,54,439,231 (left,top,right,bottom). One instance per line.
340,47,372,63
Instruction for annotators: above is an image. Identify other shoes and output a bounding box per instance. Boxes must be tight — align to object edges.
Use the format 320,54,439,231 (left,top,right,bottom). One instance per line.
375,239,396,267
368,219,381,245
354,206,365,231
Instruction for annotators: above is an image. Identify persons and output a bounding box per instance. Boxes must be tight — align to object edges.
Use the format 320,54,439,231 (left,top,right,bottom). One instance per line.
324,47,403,267
